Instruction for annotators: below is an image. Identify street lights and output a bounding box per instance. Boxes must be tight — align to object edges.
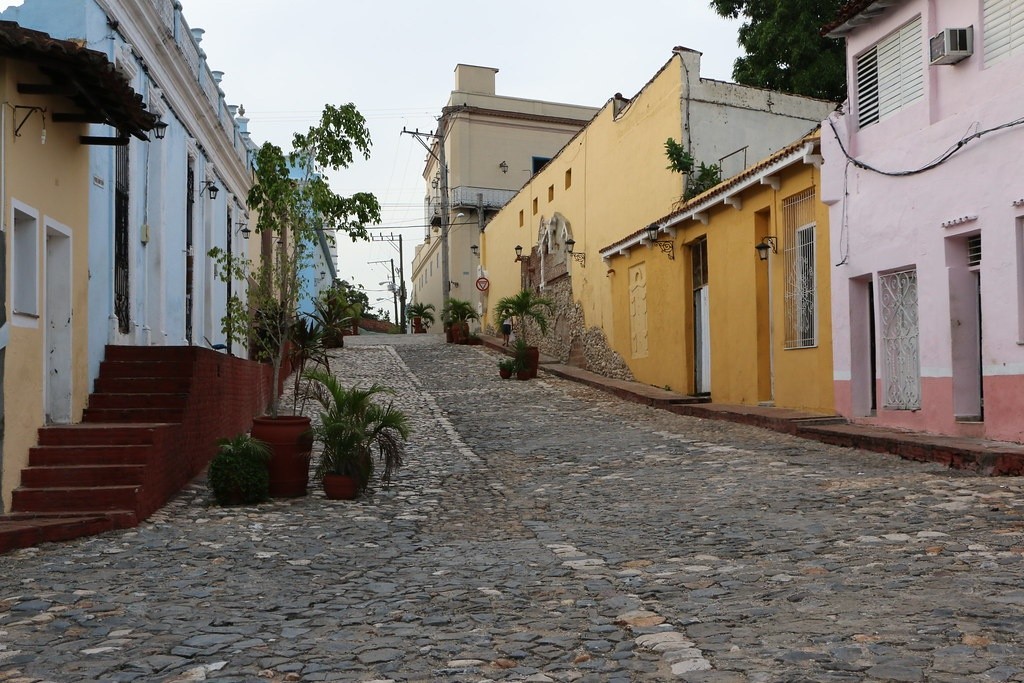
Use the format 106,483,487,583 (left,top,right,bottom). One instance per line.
378,280,406,334
376,297,398,326
441,212,465,333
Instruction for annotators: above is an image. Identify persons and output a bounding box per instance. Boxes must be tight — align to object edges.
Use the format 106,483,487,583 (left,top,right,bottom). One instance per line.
502,309,513,347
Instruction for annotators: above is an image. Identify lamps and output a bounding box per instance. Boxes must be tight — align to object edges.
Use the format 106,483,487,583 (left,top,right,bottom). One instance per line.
470,245,480,258
645,223,674,260
200,181,219,200
755,236,777,260
271,237,283,248
297,244,306,253
314,271,326,286
235,223,250,239
154,114,168,138
514,244,530,266
565,239,585,268
431,179,440,189
499,161,508,174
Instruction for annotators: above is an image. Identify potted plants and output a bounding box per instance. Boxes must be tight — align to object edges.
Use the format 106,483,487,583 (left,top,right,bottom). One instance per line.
204,101,416,507
492,288,554,380
438,298,480,343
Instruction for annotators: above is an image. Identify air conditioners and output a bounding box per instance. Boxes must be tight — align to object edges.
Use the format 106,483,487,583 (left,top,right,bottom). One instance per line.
928,27,973,66
